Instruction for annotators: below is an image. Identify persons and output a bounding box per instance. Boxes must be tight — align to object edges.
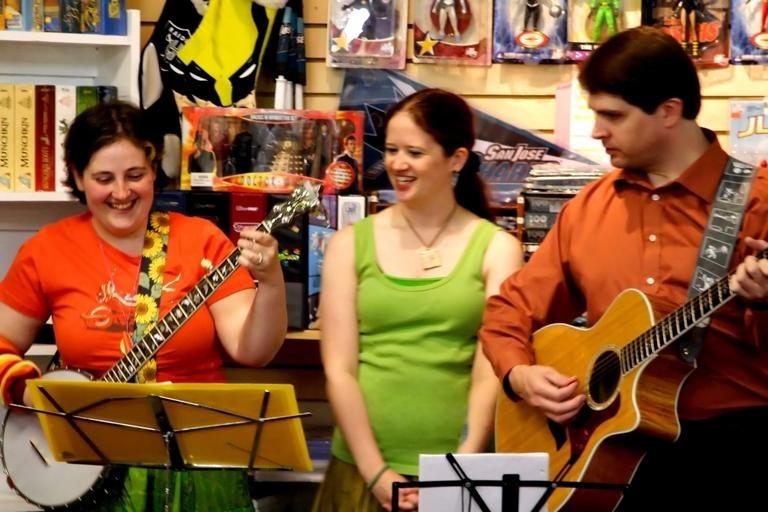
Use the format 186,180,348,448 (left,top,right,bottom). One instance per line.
0,103,288,512
339,0,768,58
311,88,524,512
335,135,358,195
478,25,768,512
189,129,214,174
341,0,768,58
209,117,332,178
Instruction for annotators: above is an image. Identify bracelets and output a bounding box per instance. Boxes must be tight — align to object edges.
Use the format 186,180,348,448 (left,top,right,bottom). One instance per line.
365,465,390,491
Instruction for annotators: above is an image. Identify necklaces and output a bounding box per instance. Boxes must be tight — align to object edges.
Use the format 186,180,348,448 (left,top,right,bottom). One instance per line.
394,202,460,269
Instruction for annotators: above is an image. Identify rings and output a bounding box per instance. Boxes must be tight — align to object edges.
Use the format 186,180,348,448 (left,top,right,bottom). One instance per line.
257,252,262,265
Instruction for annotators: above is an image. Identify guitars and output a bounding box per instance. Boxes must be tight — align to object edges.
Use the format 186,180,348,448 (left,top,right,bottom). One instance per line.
495,247,768,511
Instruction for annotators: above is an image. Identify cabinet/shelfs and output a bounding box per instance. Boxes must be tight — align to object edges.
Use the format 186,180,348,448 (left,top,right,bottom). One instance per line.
0,8,139,371
